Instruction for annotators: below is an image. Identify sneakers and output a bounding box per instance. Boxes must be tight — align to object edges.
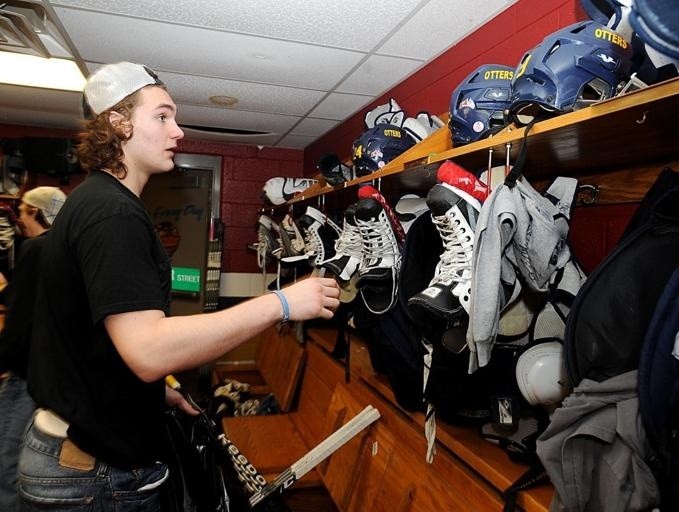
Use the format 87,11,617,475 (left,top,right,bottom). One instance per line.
406,182,483,355
255,212,315,290
354,199,404,315
305,205,343,279
315,204,363,304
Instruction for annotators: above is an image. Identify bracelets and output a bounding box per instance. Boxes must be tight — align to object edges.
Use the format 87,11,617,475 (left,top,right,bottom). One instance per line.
271,287,290,323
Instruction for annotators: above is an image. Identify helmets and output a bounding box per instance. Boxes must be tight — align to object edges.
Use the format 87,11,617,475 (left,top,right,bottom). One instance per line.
449,0,679,148
352,123,417,177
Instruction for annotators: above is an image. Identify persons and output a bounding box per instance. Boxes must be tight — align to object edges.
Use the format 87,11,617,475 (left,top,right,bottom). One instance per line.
1,185,70,508
17,60,340,510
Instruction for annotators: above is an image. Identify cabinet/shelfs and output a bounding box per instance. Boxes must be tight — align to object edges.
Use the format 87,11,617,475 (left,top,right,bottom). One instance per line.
254,76,679,511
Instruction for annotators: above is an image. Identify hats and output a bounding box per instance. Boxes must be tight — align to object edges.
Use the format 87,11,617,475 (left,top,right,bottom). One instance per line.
82,62,163,119
21,186,68,225
